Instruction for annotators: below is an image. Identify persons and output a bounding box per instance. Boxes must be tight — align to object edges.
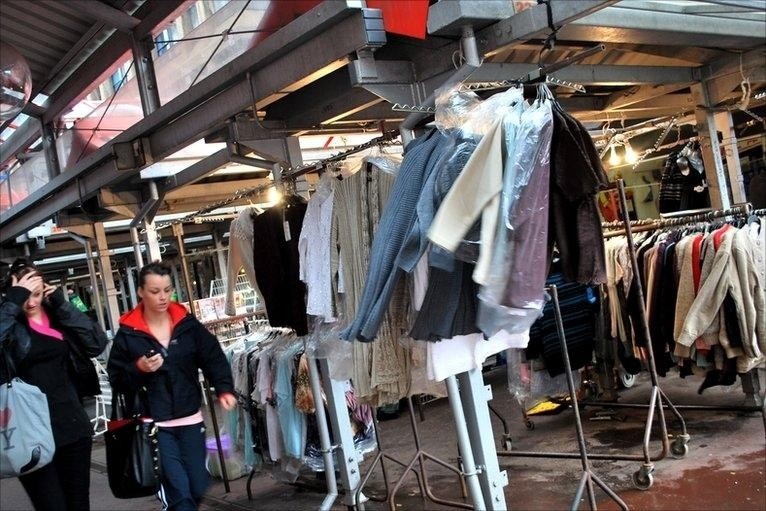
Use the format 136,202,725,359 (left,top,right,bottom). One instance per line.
0,255,109,511
106,262,239,511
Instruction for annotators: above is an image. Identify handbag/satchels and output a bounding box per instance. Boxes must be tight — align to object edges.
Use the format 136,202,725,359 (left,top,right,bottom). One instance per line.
103,414,163,501
0,376,54,480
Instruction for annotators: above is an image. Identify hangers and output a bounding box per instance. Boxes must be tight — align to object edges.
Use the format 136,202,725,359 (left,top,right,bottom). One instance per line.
519,83,564,113
228,319,296,350
601,203,766,239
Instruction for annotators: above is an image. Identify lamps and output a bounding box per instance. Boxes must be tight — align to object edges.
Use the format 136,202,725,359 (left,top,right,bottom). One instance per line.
608,140,638,168
